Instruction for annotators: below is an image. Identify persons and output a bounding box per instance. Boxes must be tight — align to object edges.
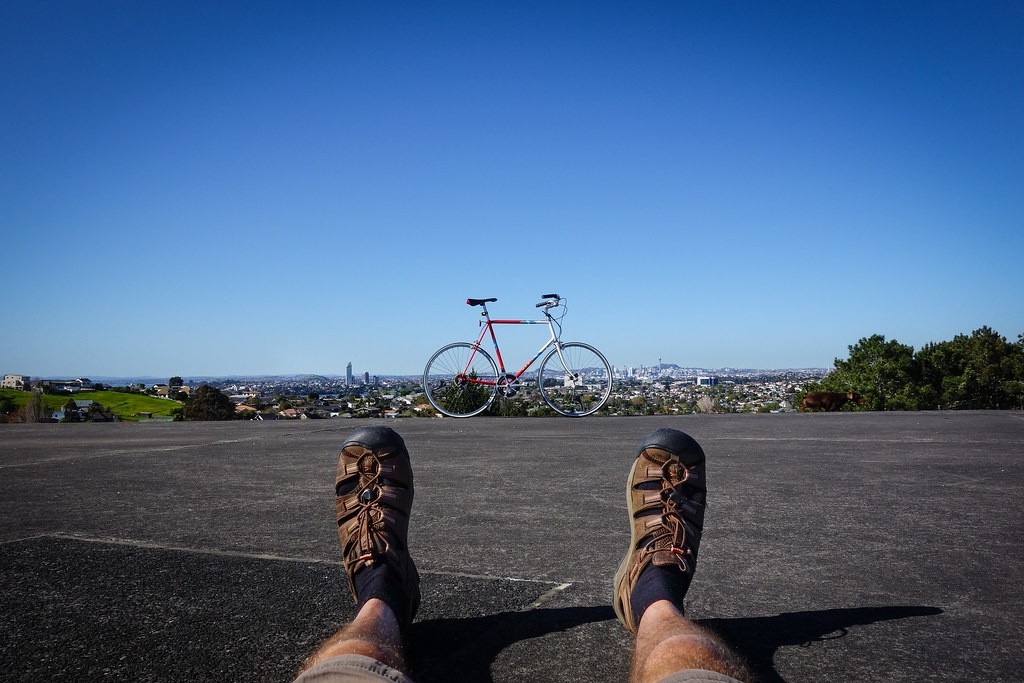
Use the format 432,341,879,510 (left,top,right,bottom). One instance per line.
293,425,757,683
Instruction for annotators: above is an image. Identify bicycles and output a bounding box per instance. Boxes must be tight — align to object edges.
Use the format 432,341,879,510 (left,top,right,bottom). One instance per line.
422,293,615,417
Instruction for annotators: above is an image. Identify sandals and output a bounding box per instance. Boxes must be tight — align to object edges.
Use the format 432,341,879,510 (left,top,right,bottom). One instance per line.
333,427,419,626
613,427,706,634
